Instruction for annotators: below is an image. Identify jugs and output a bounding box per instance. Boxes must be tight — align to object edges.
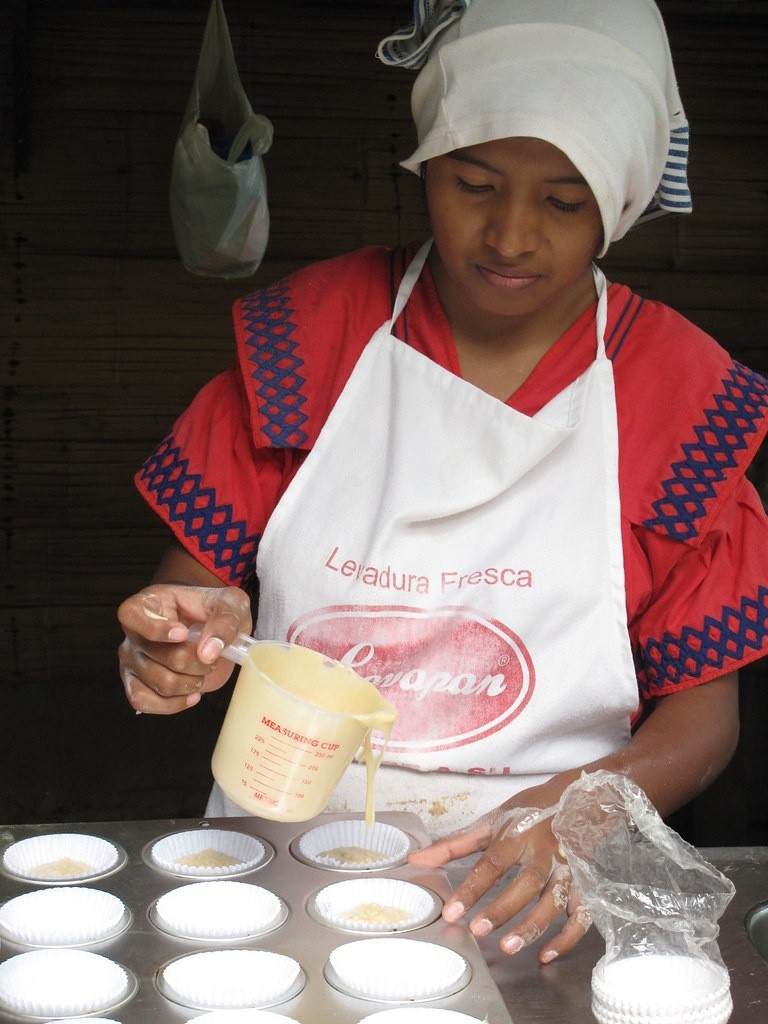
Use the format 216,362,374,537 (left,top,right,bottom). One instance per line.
182,619,398,823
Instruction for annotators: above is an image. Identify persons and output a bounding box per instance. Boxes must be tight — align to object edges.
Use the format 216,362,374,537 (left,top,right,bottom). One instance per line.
117,1,768,963
